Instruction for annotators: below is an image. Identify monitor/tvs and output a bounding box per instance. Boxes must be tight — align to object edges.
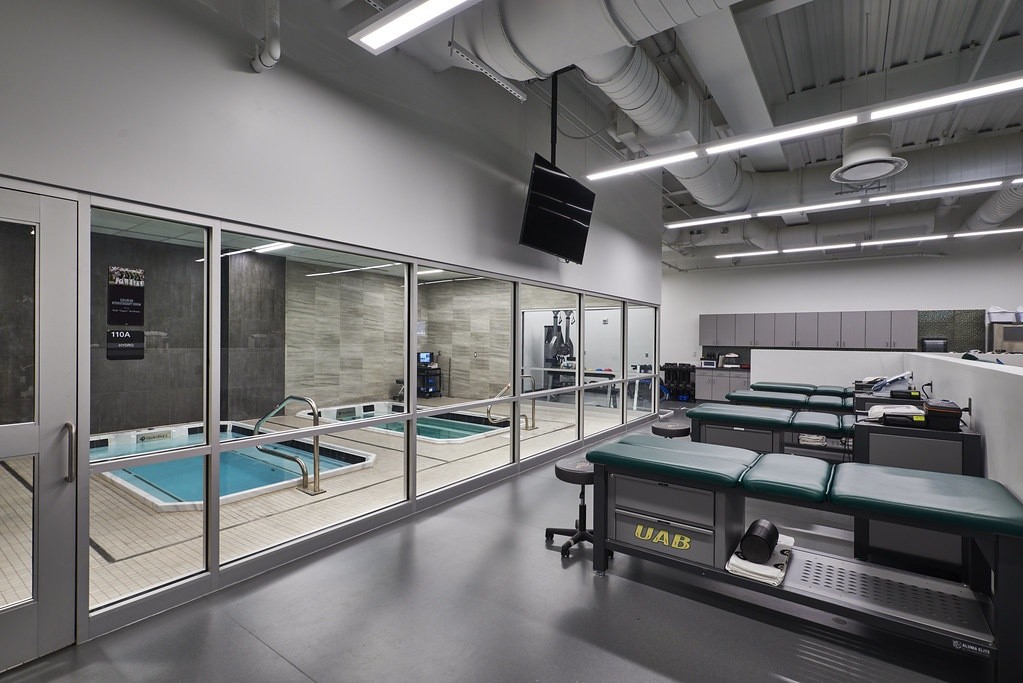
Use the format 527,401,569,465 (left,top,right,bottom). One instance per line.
520,152,595,265
417,353,433,365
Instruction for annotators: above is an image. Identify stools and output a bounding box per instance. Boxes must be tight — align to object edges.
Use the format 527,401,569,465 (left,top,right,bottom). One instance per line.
545,456,594,558
652,422,690,438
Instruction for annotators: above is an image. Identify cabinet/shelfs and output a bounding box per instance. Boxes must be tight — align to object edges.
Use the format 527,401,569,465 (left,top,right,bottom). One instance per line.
418,366,442,399
695,310,918,402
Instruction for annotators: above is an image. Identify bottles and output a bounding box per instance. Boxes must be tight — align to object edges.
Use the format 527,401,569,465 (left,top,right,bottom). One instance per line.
907,377,913,390
